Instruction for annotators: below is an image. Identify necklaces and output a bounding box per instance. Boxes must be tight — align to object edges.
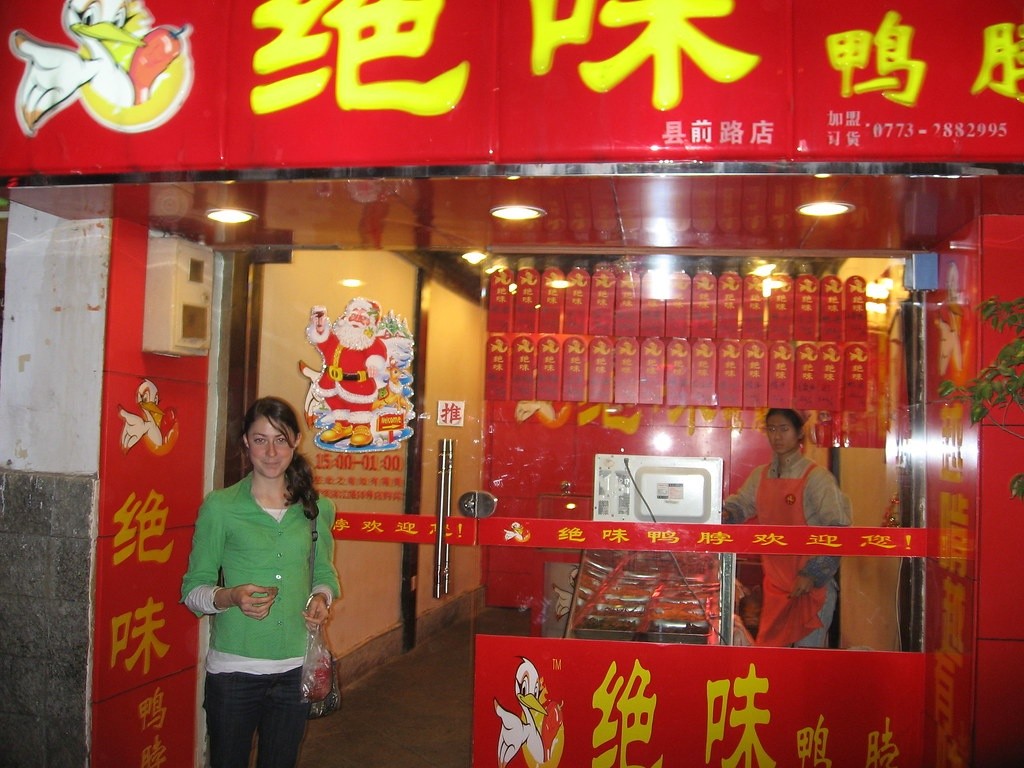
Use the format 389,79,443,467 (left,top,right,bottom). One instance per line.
255,492,285,521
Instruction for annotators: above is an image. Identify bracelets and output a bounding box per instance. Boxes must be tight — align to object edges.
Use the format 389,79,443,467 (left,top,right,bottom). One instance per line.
211,589,230,613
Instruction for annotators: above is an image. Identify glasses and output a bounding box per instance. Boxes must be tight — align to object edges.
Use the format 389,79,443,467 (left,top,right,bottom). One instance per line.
766,425,798,432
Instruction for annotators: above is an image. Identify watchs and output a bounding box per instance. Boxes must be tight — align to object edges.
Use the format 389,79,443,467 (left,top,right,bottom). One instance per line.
304,595,331,612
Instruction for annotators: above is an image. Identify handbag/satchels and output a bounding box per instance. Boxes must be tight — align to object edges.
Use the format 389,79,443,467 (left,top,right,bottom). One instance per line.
300,621,340,718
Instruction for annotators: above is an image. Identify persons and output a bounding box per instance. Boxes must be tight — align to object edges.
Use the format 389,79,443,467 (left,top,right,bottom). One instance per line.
719,408,850,649
179,397,340,768
